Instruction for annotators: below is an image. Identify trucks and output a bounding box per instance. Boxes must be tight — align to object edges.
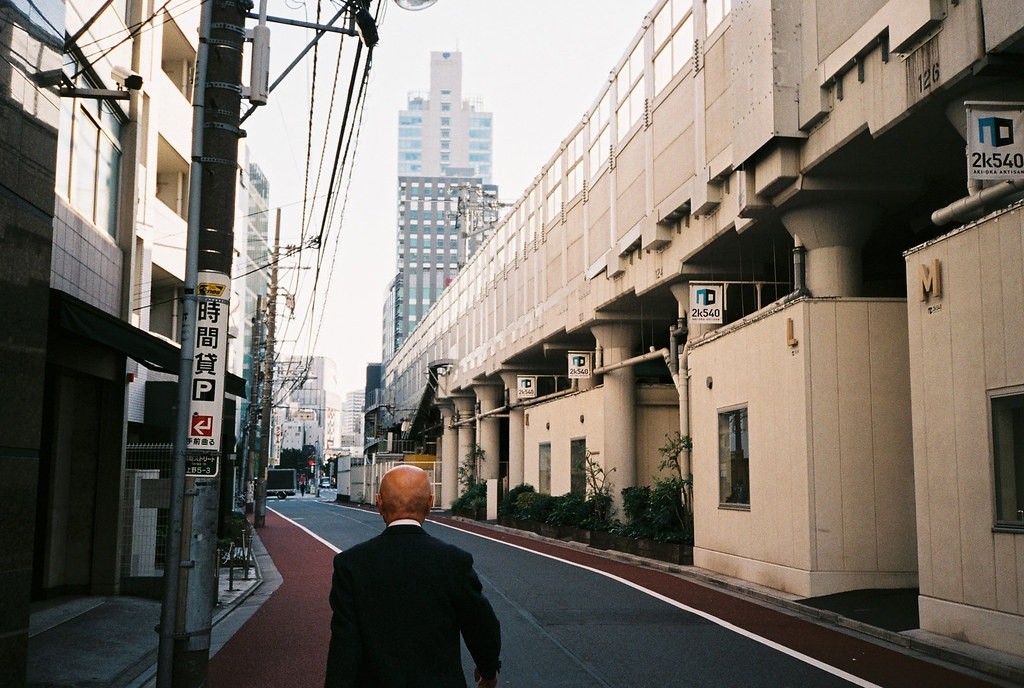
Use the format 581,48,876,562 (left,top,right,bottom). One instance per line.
252,467,296,499
320,477,331,488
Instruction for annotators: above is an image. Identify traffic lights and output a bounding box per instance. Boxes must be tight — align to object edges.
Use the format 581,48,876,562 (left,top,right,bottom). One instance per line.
307,454,316,467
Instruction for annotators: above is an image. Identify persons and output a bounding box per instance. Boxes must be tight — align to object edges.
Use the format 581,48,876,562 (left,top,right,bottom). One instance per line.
331,475,335,488
325,465,501,688
300,482,306,496
